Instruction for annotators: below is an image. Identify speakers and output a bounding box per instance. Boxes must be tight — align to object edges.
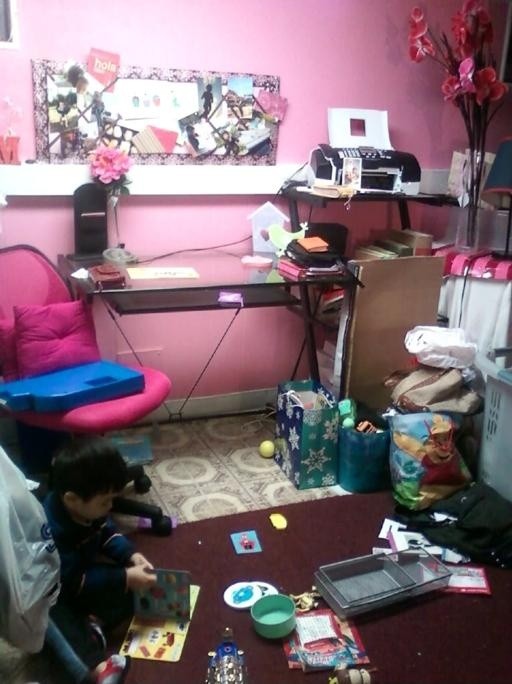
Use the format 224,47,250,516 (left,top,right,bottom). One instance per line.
68,182,108,260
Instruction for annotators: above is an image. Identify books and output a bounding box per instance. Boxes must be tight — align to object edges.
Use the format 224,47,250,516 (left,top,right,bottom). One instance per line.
279,259,345,278
283,608,370,672
354,227,433,260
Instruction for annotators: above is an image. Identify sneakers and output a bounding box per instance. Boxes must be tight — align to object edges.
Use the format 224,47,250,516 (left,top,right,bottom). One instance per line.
87,613,108,650
95,654,129,684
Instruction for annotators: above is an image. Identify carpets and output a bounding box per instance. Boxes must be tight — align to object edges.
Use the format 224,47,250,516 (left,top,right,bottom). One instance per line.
47,486,512,684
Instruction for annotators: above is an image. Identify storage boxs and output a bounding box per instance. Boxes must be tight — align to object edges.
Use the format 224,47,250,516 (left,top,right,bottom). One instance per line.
474,346,512,501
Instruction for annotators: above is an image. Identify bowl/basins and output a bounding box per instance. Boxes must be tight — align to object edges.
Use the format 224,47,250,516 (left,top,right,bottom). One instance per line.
250,593,297,640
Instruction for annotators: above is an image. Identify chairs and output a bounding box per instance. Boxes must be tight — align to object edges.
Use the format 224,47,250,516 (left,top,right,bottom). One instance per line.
0,244,174,475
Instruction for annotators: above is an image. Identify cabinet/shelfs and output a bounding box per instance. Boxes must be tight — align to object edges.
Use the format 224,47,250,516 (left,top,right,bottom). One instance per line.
282,181,459,334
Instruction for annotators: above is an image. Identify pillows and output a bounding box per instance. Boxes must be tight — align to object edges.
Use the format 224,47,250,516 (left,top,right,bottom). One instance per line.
12,298,101,378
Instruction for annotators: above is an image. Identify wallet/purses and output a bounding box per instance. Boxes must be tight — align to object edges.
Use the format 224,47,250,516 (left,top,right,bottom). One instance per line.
89,263,125,290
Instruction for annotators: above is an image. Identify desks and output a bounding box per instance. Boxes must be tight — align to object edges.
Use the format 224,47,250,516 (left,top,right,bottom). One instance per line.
58,250,351,422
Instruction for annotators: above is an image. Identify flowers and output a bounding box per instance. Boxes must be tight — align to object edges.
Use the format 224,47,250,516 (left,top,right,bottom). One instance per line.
91,143,133,248
408,0,512,250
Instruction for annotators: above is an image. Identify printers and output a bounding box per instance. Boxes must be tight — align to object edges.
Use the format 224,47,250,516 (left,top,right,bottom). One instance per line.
306,107,421,195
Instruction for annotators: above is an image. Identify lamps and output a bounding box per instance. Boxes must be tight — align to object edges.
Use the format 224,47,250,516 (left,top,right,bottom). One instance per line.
480,135,512,259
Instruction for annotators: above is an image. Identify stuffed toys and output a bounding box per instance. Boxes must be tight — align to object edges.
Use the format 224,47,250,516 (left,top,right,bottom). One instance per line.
328,668,371,684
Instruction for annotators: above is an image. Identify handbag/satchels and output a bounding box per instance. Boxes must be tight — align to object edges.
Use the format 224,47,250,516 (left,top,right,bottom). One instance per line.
285,238,342,268
338,399,390,493
394,479,511,569
389,412,471,512
274,379,340,489
383,367,485,415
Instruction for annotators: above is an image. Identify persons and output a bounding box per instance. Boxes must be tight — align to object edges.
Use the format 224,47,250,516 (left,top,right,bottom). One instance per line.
41,432,159,655
52,64,262,160
347,160,358,182
